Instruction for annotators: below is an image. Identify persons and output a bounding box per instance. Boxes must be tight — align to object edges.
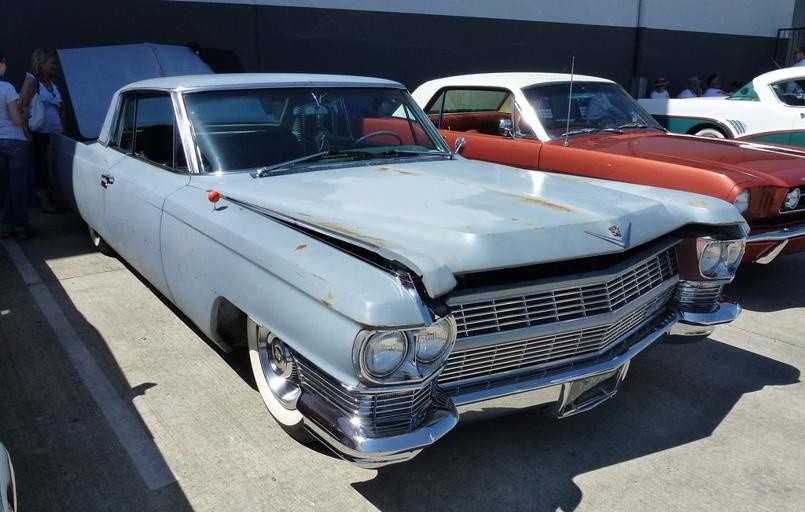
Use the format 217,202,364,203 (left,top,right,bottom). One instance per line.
0,54,35,236
265,96,283,123
19,48,74,218
366,96,391,118
703,73,733,98
650,78,671,100
677,77,704,99
785,48,805,95
586,91,629,129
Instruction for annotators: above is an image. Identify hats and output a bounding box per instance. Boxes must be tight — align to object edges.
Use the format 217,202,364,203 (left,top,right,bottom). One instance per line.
688,76,703,84
648,77,669,86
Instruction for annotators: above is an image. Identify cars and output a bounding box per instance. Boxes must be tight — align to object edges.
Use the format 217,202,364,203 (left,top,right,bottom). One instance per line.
49,44,750,469
361,72,804,269
635,64,804,150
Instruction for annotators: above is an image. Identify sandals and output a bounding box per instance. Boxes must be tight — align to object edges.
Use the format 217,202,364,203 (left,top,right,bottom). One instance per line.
0,229,27,241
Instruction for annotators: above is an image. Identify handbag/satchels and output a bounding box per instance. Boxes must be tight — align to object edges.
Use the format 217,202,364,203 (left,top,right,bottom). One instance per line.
18,75,44,132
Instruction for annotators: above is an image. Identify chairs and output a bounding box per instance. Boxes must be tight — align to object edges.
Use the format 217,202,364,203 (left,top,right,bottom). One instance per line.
298,101,340,160
